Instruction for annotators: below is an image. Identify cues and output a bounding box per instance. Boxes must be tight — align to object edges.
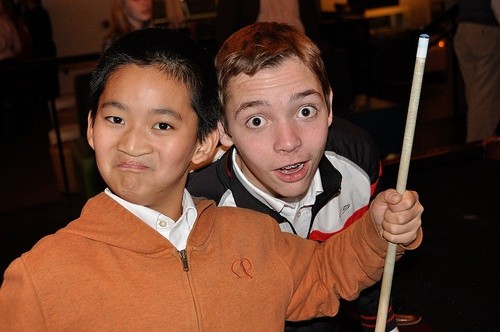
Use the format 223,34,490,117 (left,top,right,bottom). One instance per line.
374,33,431,332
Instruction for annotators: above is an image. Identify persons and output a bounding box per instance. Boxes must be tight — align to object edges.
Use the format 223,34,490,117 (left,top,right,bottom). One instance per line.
0,29,425,332
0,0,500,332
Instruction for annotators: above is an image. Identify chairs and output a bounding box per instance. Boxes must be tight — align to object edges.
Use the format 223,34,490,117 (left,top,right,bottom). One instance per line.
0,45,66,169
